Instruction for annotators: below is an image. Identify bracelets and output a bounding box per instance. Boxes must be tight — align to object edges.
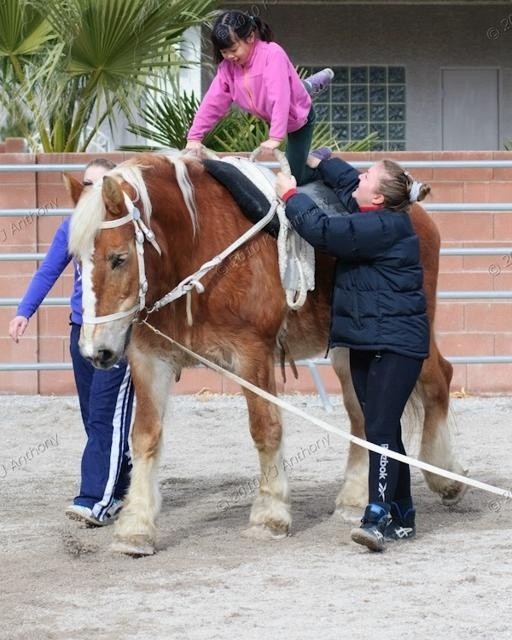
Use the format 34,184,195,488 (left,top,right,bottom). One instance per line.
279,187,297,203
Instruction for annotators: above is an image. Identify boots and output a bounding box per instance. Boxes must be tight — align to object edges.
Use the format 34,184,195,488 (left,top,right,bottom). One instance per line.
351,502,393,553
385,501,417,539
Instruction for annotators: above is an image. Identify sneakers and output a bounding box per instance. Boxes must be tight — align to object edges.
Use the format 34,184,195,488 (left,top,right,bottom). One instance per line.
66,501,123,525
311,145,330,159
306,69,334,96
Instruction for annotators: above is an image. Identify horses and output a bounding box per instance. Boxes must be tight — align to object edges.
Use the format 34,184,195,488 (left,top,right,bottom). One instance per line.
60,148,470,557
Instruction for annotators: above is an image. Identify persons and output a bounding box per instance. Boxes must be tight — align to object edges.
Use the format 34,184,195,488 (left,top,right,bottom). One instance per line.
271,143,431,553
6,156,139,530
177,8,334,190
